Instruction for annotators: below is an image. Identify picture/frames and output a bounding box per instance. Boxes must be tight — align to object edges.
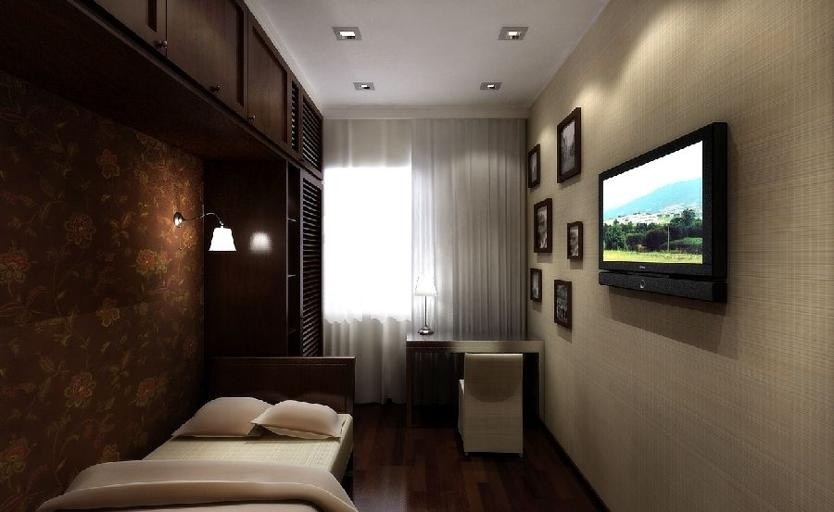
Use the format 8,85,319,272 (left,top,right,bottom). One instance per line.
567,221,584,262
530,268,542,302
554,278,572,330
533,197,552,253
557,106,581,183
528,143,541,189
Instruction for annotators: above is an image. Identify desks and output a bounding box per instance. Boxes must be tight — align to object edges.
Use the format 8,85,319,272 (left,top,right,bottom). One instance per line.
407,335,546,428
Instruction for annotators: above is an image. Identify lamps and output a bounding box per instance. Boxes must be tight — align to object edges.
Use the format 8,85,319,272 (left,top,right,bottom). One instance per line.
173,207,236,253
414,273,438,335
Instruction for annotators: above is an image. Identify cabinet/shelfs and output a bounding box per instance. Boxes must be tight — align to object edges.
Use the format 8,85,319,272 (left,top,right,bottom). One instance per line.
1,1,244,113
206,64,325,396
244,7,295,161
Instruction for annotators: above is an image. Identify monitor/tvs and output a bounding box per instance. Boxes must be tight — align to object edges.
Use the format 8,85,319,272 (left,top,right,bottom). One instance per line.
598,122,728,279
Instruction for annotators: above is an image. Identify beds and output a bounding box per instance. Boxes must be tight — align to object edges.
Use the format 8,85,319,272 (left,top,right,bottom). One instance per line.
98,356,359,511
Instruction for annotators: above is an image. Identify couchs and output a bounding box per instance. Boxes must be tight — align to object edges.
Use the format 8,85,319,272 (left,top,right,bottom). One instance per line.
454,351,524,462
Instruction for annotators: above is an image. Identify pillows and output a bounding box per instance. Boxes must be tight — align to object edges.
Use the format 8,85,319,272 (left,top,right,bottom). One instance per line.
168,395,273,438
249,399,346,439
